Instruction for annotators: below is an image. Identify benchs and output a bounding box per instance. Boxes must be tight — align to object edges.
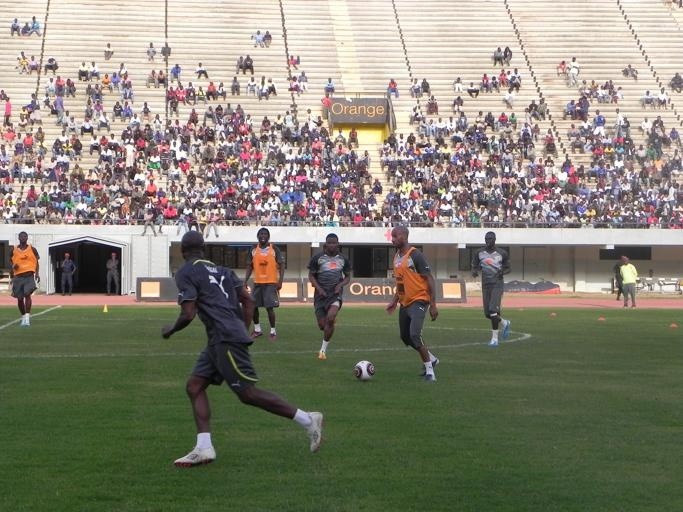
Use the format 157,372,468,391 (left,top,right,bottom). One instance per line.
0,1,683,225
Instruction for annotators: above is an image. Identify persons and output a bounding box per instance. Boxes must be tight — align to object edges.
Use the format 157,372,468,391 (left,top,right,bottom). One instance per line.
160,230,328,470
1,75,151,139
613,262,625,300
105,253,120,295
11,16,43,41
306,233,352,360
7,233,43,324
642,72,682,109
58,251,77,296
164,61,240,117
469,231,512,347
387,77,439,126
243,227,285,339
235,29,336,111
385,227,440,380
374,112,682,228
77,41,168,101
524,55,640,123
451,45,521,115
2,89,385,239
619,255,638,309
16,52,59,77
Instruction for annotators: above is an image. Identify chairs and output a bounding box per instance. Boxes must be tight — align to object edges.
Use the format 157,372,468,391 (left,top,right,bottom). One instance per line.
318,351,326,360
268,333,276,341
174,446,216,467
421,358,440,382
306,412,323,452
250,330,263,338
21,313,31,326
488,320,511,346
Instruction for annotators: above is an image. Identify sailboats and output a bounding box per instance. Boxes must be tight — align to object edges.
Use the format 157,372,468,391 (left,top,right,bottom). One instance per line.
352,361,376,380
